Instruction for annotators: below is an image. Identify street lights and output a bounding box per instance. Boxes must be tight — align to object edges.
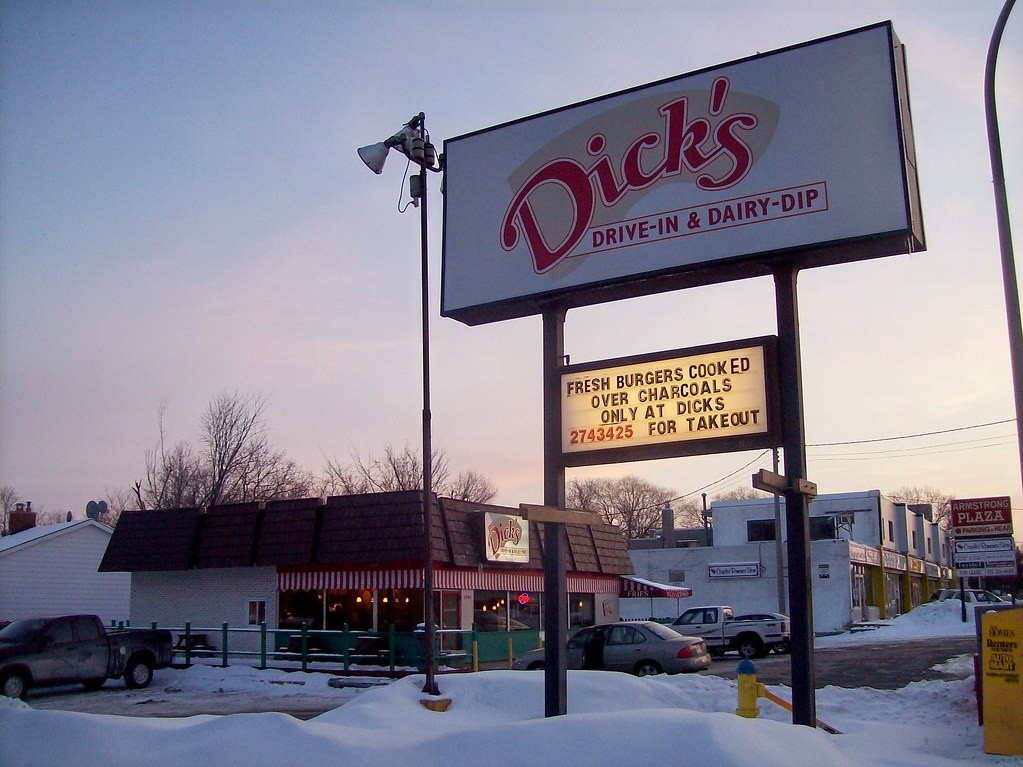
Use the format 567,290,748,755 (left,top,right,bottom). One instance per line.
357,110,443,694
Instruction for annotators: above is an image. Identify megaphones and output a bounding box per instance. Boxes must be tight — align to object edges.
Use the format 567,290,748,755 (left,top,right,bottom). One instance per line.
392,126,415,153
357,142,388,174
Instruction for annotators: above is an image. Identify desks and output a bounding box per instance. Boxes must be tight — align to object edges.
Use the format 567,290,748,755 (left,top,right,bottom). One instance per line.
353,635,381,654
288,634,314,651
175,632,208,651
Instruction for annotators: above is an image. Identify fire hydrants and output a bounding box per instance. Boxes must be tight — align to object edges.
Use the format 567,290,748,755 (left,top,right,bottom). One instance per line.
734,654,765,719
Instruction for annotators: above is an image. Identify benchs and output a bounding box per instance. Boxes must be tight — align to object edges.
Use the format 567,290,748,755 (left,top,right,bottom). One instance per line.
348,648,392,667
172,645,216,658
278,647,321,662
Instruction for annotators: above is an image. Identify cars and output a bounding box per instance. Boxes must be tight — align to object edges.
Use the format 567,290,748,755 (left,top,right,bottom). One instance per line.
928,587,1011,606
443,608,532,631
510,620,712,678
736,611,790,647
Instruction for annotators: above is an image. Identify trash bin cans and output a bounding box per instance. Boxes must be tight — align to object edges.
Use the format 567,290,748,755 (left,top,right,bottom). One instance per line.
416,624,440,671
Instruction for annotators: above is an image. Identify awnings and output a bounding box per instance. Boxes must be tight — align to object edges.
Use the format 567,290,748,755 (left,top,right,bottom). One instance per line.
619,577,692,617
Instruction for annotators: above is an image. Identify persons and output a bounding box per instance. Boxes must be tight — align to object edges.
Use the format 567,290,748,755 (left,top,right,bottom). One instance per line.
584,628,605,670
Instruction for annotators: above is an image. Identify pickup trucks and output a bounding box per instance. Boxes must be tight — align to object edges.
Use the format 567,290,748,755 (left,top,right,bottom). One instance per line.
0,612,173,702
661,605,790,659
